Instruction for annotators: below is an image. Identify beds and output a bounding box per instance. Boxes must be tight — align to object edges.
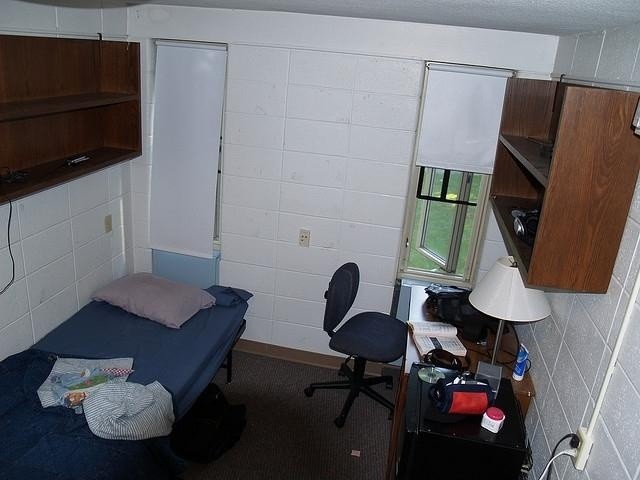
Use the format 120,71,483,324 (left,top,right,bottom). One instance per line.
0,272,253,480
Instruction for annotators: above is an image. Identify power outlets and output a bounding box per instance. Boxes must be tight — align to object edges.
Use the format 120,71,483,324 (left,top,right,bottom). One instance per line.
299,230,310,248
571,427,593,470
105,215,112,233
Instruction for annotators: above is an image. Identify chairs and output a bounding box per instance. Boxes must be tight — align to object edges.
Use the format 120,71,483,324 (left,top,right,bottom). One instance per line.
302,262,408,429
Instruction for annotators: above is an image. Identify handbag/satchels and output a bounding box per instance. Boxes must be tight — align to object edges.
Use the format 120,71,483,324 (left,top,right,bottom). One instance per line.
429,376,495,416
423,284,510,342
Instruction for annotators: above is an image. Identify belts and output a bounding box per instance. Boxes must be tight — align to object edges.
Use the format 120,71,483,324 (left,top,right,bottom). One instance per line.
425,348,472,373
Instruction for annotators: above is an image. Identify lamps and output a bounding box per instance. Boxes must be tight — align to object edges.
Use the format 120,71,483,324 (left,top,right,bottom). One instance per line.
469,255,552,365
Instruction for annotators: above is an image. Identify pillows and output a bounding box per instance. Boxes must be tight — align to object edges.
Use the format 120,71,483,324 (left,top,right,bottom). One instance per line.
90,272,216,330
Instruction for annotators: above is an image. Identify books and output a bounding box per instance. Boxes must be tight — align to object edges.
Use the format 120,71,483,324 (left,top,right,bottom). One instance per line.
406,318,468,357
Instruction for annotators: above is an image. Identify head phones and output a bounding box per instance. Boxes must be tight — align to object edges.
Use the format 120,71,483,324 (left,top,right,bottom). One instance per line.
513,215,539,245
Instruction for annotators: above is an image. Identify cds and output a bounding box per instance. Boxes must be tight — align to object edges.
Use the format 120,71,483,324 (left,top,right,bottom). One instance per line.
418,367,445,384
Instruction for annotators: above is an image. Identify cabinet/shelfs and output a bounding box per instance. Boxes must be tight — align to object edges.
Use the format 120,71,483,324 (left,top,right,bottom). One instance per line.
1,34,143,203
487,78,639,294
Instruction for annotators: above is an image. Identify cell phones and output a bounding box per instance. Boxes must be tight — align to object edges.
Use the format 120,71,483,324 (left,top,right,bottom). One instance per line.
64,151,90,165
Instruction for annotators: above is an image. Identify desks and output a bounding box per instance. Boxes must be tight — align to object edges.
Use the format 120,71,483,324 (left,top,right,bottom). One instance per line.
387,363,530,479
385,277,536,478
